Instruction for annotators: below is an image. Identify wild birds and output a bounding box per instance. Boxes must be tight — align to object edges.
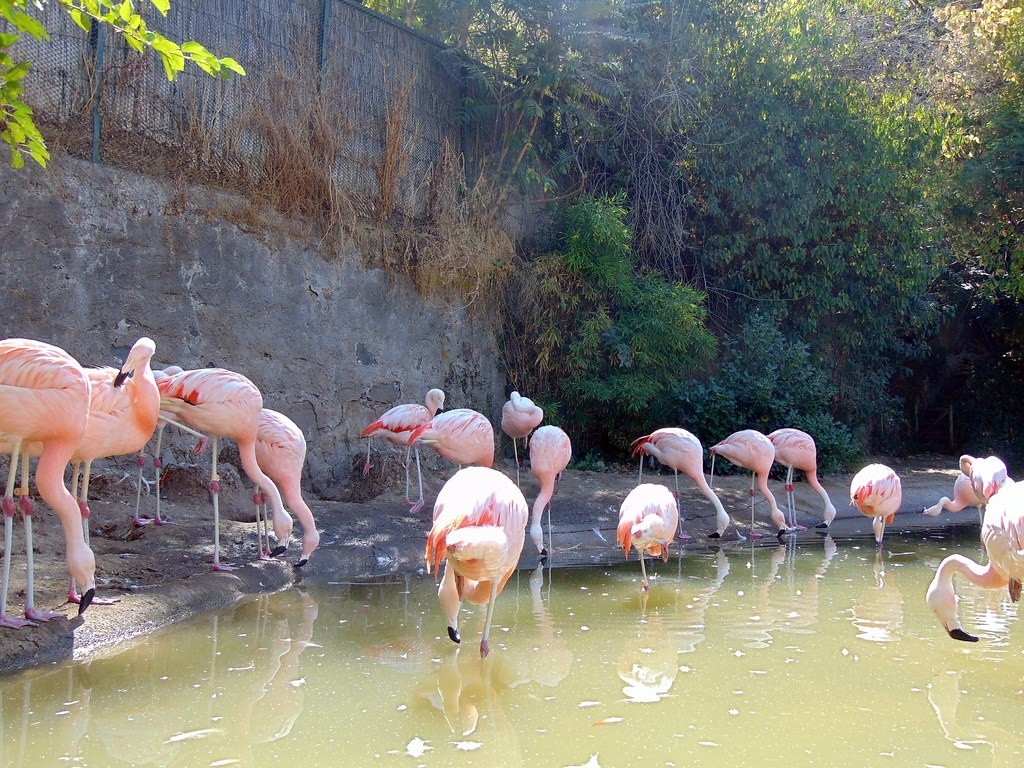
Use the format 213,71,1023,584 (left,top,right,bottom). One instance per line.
849,462,902,547
358,388,573,661
709,427,837,538
0,334,320,631
922,451,1024,642
614,427,730,592
